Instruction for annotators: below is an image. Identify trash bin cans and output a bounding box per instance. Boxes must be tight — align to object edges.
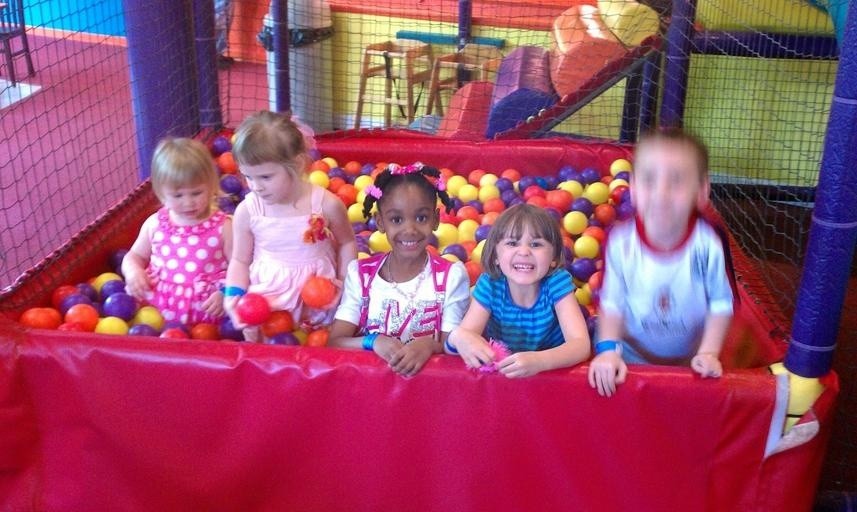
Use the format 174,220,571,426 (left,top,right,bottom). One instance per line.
262,0,332,131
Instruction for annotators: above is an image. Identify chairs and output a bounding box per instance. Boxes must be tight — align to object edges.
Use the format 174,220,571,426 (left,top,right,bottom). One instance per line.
0,0,35,86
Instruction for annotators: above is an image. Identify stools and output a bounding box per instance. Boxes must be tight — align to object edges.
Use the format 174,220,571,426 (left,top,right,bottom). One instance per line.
425,39,505,121
351,37,443,130
